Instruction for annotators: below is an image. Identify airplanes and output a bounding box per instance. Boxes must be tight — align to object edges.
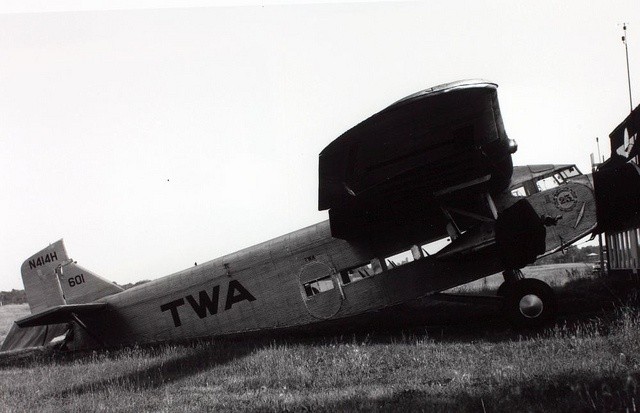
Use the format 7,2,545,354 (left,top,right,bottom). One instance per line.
0,160,606,369
